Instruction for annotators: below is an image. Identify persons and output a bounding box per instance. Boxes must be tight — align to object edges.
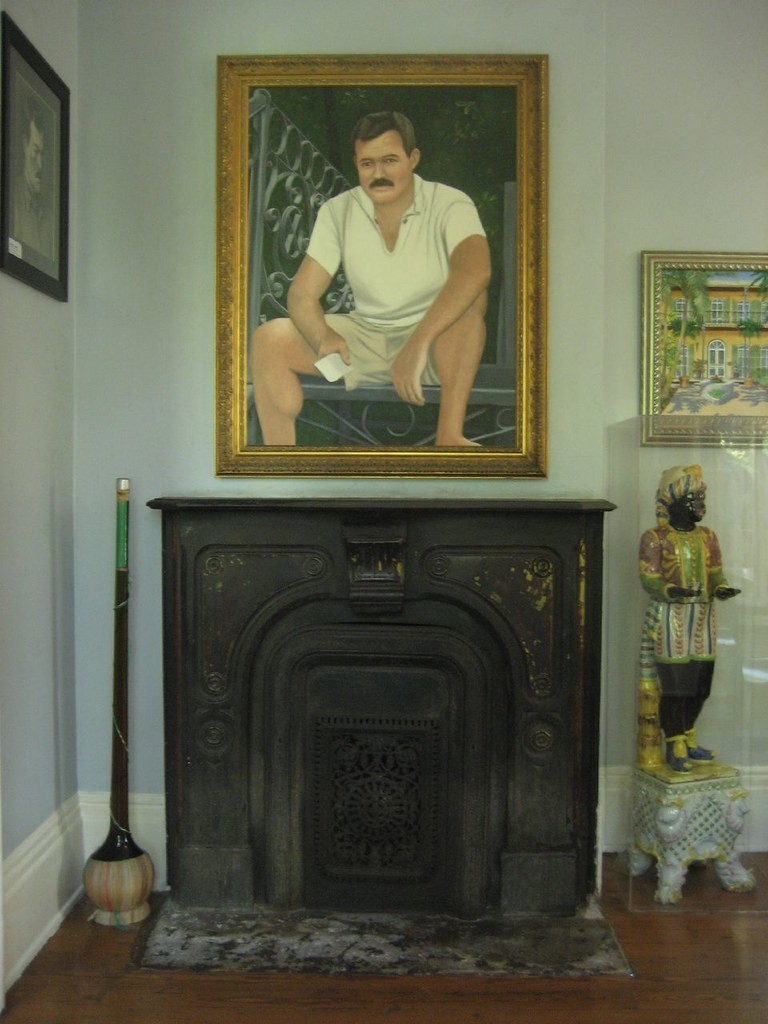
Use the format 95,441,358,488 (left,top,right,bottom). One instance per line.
638,462,742,772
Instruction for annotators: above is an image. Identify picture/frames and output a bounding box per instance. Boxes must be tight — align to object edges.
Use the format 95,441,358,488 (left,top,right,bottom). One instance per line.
0,8,71,304
640,251,767,448
214,54,548,480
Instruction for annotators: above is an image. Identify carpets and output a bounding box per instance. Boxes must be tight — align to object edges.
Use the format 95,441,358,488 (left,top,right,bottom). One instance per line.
128,901,636,978
